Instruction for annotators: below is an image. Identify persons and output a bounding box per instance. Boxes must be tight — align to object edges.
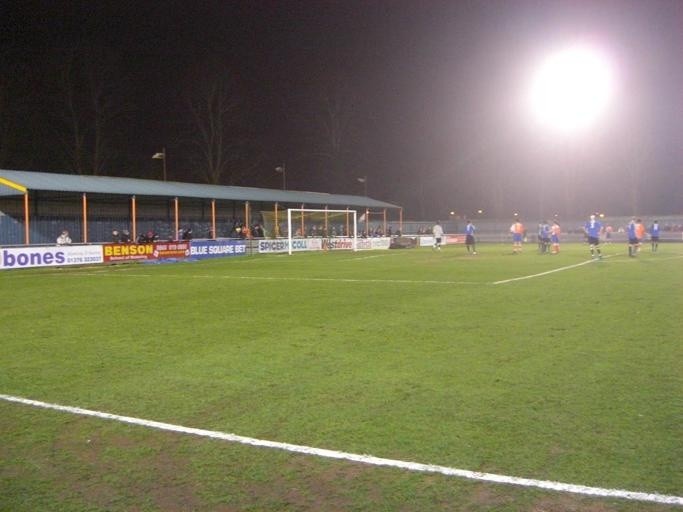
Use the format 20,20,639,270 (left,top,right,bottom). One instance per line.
510,218,525,253
463,219,477,254
56,229,72,247
432,219,444,250
536,210,683,260
